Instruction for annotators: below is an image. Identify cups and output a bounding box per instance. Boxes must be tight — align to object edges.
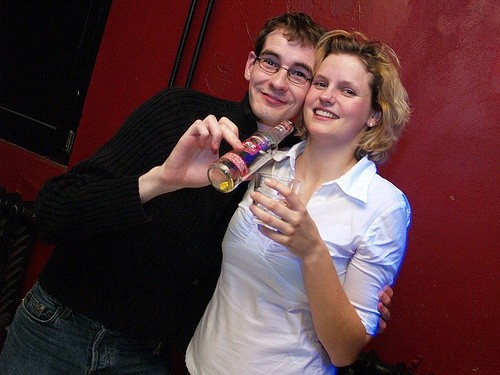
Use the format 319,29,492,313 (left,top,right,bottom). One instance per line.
249,172,302,225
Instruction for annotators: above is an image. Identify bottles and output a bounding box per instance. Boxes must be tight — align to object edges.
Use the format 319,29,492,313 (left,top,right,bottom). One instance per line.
206,119,295,194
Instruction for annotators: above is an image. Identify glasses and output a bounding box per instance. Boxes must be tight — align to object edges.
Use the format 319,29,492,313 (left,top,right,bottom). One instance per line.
253,57,313,85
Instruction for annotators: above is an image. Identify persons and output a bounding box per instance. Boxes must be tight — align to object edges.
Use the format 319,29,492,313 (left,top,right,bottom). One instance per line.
0,12,396,375
179,28,412,375
